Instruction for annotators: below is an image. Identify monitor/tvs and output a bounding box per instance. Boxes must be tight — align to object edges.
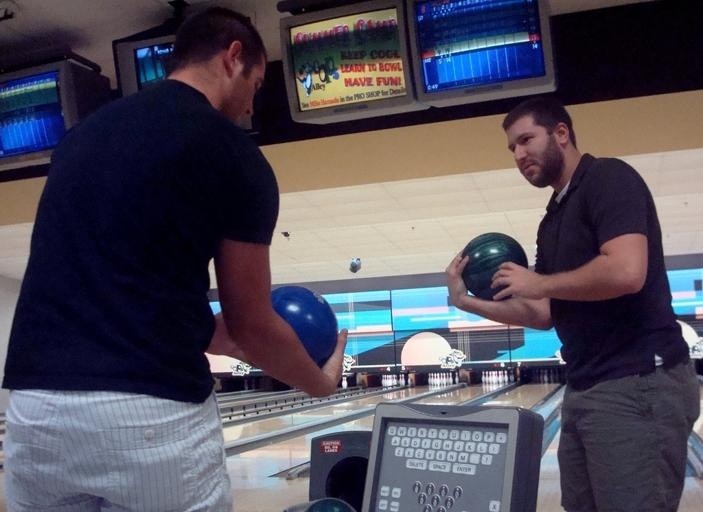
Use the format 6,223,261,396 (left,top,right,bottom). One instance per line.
0,60,111,172
279,0,432,125
116,32,252,130
407,0,559,108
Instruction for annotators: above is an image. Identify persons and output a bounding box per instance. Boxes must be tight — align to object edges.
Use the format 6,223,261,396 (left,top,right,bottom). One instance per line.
445,97,700,512
0,4,348,512
